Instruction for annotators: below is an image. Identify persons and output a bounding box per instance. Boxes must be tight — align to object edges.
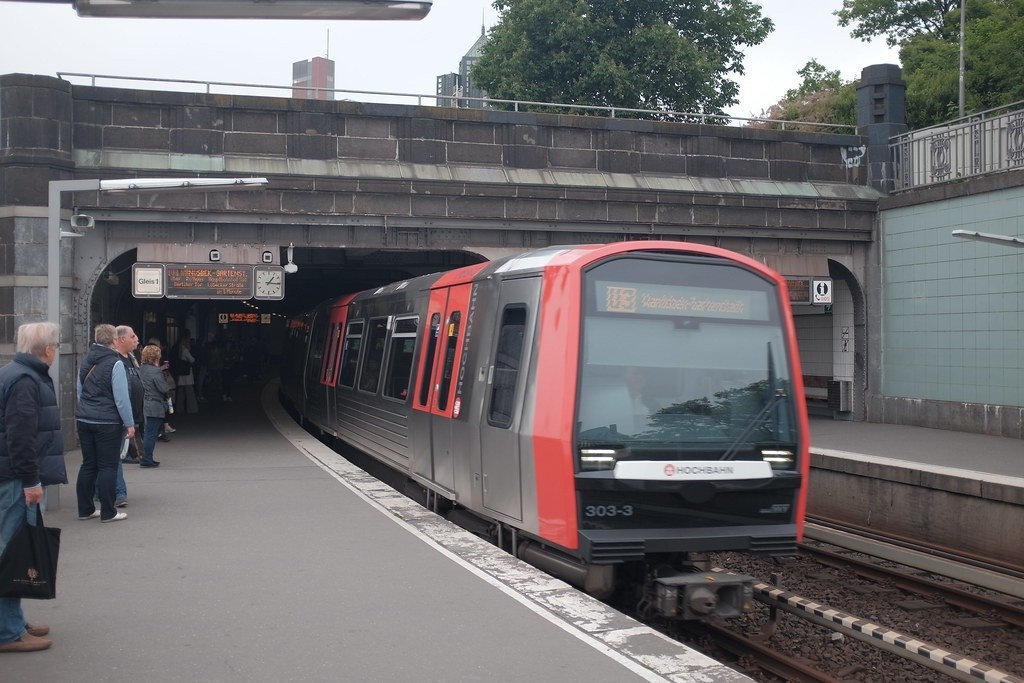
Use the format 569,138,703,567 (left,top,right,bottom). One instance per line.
188,329,257,404
93,324,195,508
0,321,70,652
76,323,136,522
363,356,381,393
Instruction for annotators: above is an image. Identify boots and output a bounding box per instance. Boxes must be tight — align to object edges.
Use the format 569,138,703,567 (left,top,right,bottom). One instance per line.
164,423,176,434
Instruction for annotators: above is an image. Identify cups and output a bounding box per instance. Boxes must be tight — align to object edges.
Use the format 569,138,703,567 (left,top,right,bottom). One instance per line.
164,361,169,364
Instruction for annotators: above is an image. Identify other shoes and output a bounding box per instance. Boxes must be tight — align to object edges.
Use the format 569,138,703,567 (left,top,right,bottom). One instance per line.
157,435,170,442
115,497,128,507
24,623,49,636
223,395,233,402
197,396,207,403
0,628,52,652
140,461,160,467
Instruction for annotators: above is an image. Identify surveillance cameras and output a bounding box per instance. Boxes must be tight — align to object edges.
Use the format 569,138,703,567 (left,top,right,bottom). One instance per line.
210,251,220,260
263,253,273,263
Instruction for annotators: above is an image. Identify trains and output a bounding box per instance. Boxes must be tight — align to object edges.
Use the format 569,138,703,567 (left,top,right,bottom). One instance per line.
282,239,811,621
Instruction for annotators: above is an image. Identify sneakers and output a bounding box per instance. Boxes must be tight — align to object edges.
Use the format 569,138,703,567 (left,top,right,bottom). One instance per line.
101,511,127,522
80,509,101,520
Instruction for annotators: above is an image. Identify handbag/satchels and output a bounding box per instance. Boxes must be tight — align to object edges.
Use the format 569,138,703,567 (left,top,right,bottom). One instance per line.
121,427,144,462
173,348,192,376
167,398,174,414
0,499,61,600
